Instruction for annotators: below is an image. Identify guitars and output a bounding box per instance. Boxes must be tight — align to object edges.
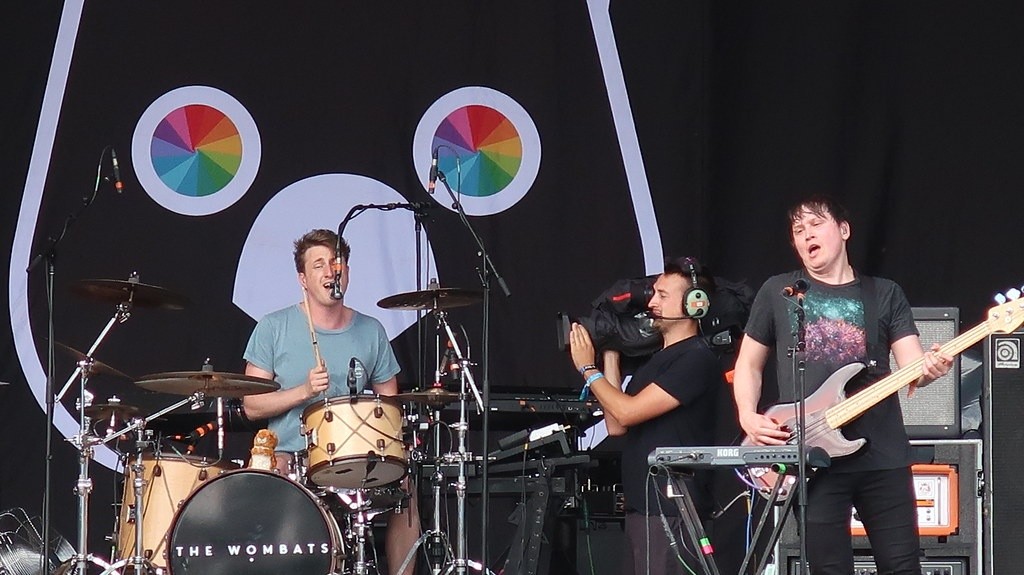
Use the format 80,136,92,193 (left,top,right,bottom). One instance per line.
728,285,1024,507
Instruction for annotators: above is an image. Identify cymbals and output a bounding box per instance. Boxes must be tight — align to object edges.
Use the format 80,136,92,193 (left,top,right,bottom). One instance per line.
377,283,484,310
35,333,136,382
132,364,281,398
390,387,483,407
65,279,172,310
80,403,168,424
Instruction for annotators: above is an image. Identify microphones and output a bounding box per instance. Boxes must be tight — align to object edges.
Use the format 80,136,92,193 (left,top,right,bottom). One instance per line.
781,278,812,297
439,332,460,379
111,148,123,194
331,249,343,300
416,421,438,447
428,148,439,194
217,396,225,459
188,421,217,437
346,358,356,386
648,309,704,321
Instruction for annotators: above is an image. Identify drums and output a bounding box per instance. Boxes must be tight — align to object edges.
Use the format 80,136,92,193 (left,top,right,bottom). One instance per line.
166,468,345,575
116,451,244,575
300,394,408,491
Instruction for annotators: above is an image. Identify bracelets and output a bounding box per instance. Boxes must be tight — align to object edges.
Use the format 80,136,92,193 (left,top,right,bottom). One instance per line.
579,365,605,401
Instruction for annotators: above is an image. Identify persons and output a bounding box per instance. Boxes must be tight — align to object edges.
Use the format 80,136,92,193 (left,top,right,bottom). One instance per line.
243,228,420,575
734,191,954,575
570,261,720,575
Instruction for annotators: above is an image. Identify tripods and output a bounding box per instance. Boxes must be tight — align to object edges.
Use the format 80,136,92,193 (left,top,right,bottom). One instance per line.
332,199,495,575
53,284,205,575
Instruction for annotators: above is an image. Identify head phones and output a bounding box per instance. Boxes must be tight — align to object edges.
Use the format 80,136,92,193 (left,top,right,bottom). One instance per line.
679,255,712,319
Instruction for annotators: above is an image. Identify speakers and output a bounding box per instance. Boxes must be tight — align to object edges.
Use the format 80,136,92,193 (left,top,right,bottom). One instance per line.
984,329,1024,575
558,514,714,575
887,305,961,441
